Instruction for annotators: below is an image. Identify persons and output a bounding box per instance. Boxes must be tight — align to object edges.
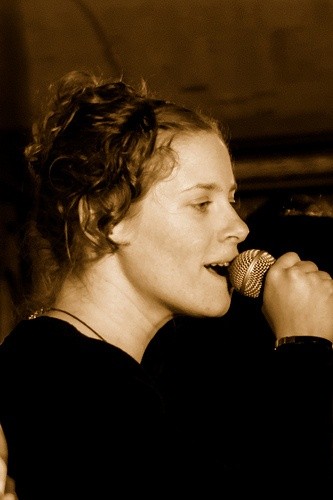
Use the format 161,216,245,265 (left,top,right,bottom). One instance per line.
0,82,333,500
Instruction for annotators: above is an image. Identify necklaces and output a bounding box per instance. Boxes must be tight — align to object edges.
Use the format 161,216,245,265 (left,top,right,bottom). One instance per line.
47,308,113,343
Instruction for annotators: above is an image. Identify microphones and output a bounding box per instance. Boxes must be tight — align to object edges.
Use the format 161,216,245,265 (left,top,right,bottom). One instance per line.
228,248,277,302
